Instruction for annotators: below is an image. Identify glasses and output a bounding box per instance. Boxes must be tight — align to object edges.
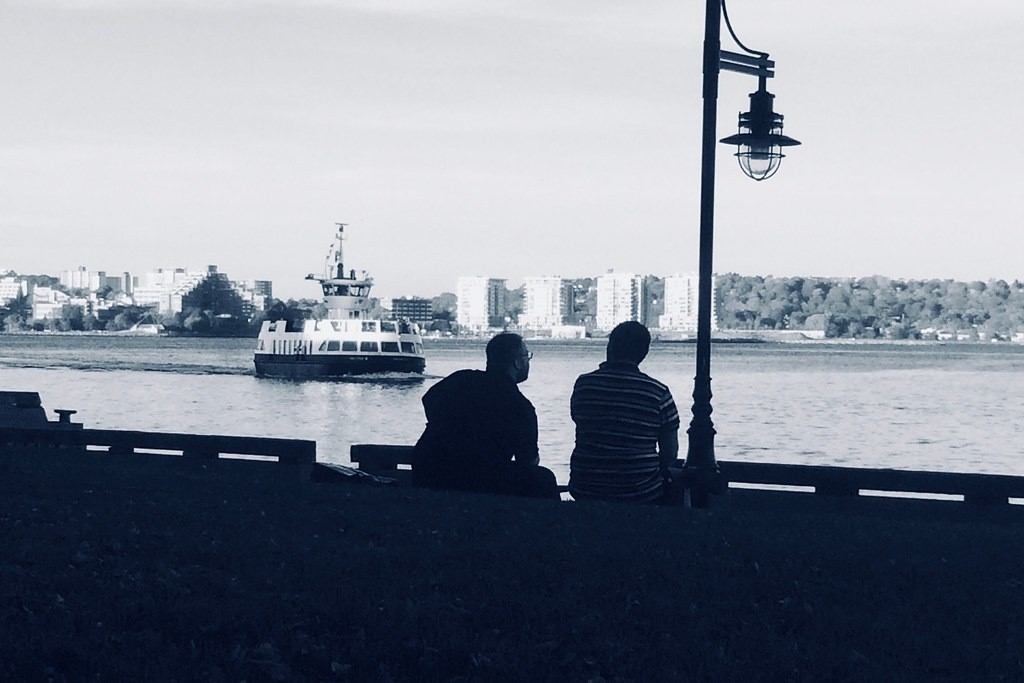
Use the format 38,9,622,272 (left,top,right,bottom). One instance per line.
517,352,533,360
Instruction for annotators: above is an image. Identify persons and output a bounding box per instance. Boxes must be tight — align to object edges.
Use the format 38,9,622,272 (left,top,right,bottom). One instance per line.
410,330,563,500
568,321,681,506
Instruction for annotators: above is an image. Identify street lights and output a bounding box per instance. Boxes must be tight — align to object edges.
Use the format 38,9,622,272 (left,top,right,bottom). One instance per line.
679,0,805,498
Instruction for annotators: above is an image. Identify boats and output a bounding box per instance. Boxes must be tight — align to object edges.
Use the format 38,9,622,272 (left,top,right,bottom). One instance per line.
254,225,428,384
